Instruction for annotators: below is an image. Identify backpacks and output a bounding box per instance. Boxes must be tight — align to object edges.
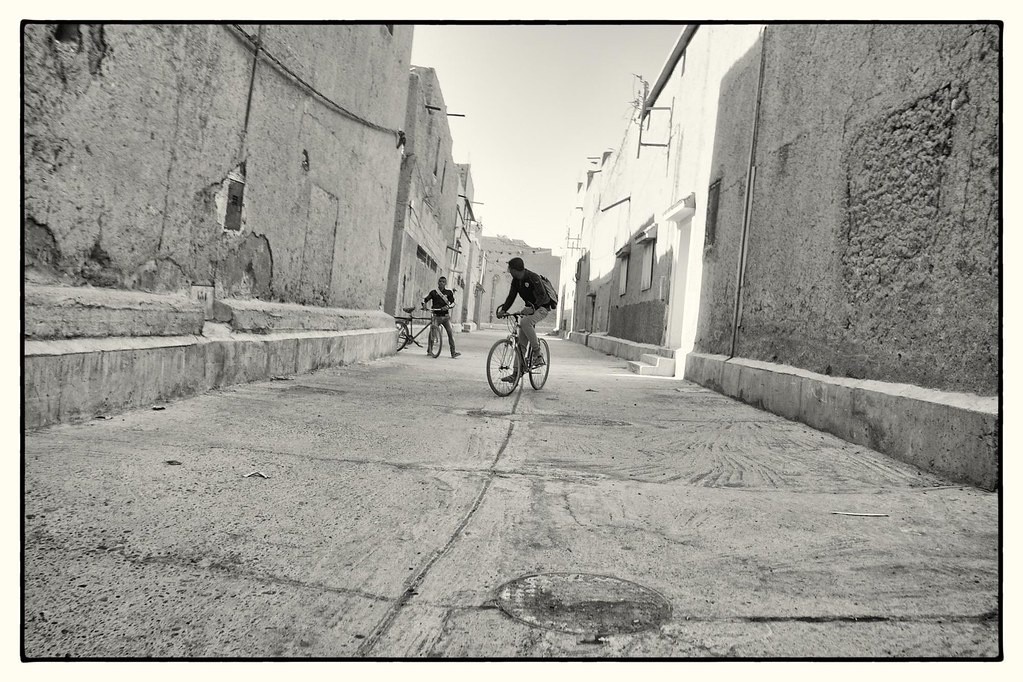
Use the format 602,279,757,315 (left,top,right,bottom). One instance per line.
526,268,558,310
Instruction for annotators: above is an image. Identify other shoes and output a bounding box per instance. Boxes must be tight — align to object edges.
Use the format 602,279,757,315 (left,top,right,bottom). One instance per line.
532,352,543,365
426,351,434,355
451,351,461,358
501,374,516,382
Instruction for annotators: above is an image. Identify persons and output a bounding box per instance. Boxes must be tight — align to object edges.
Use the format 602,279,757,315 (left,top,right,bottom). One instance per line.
421,276,461,359
496,257,552,383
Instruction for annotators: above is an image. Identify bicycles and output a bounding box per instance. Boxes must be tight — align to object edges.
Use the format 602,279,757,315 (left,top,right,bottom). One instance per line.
395,303,446,358
487,300,551,397
